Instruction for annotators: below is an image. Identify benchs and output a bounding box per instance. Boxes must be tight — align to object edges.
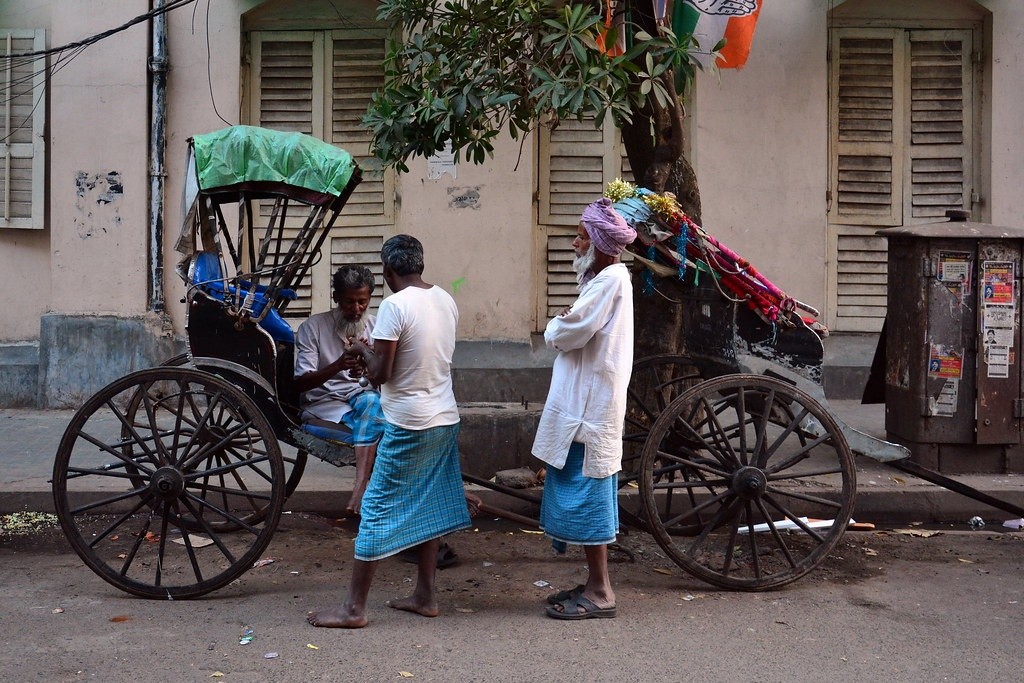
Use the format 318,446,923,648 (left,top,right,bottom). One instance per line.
183,250,295,423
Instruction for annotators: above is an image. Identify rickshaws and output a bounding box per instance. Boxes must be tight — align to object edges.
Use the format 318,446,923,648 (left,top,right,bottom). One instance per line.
613,184,1024,595
47,122,637,601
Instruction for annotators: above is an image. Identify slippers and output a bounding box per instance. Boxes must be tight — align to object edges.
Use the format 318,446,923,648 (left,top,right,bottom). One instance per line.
402,546,458,567
545,595,617,620
547,582,585,602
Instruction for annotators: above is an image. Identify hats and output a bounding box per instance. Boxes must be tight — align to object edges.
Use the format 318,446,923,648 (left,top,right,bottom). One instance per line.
582,196,636,256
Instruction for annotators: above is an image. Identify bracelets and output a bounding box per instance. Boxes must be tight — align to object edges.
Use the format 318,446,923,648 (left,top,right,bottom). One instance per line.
362,351,374,360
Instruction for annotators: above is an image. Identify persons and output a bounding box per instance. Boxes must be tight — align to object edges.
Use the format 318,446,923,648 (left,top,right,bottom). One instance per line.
307,234,471,627
531,199,634,621
295,264,481,521
